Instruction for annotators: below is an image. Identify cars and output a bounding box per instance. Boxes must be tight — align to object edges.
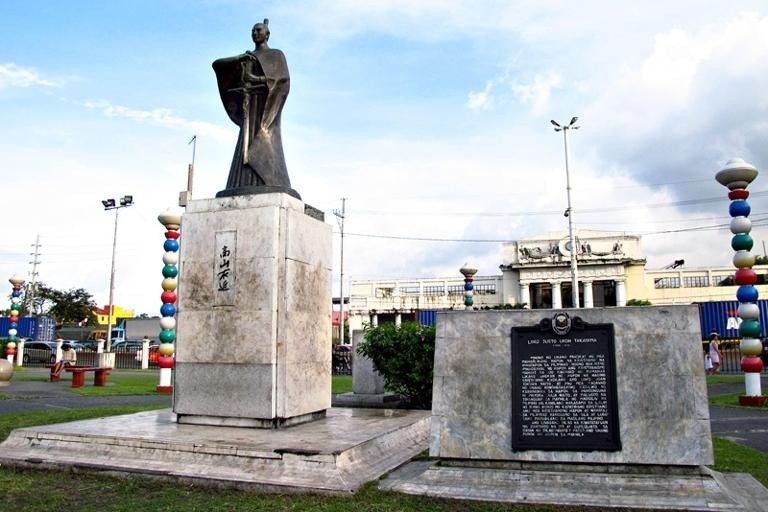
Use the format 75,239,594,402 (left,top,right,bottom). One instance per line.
23,330,176,365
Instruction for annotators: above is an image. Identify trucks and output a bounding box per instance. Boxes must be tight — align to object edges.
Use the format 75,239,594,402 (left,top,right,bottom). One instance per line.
111,319,163,346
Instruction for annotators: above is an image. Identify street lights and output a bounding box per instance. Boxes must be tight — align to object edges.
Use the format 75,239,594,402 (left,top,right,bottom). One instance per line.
551,116,582,307
101,194,134,368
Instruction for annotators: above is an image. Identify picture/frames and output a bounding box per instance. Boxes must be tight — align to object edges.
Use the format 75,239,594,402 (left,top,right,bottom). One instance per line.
509,312,622,452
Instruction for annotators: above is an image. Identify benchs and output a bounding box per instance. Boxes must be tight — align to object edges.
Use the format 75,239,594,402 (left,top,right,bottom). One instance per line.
44,364,113,386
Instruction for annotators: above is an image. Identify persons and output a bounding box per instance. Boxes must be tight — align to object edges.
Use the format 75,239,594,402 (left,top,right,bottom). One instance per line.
704,328,723,374
212,18,301,200
51,344,77,377
